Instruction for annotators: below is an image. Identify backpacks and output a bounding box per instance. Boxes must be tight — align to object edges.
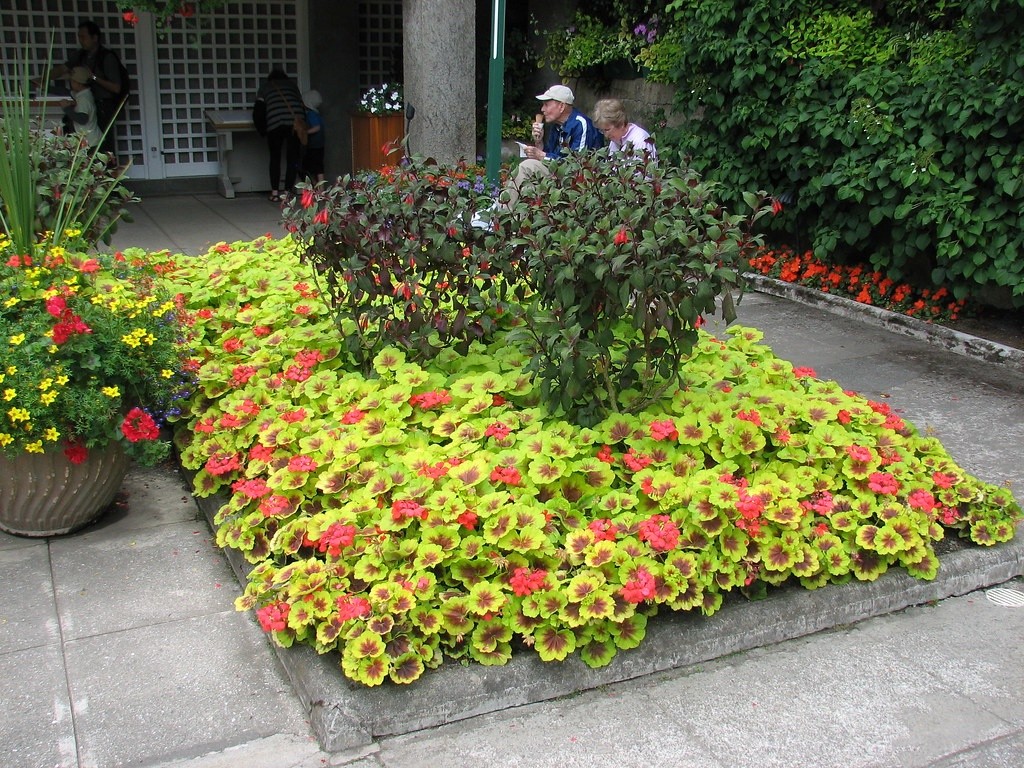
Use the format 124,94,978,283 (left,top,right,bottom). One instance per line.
80,49,130,98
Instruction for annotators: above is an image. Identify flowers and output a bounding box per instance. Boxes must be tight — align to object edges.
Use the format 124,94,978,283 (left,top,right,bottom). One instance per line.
115,0,232,51
0,172,198,464
357,81,404,116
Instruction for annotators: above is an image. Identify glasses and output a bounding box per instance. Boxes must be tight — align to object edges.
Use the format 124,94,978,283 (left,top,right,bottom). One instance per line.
598,125,615,135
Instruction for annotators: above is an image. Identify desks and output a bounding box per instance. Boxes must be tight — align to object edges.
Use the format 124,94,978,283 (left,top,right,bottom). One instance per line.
350,111,404,179
203,110,256,198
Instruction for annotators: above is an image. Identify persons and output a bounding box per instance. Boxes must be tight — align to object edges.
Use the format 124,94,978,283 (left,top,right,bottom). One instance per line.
253,68,306,202
593,99,658,202
30,20,130,207
61,65,106,192
301,90,326,196
457,85,599,234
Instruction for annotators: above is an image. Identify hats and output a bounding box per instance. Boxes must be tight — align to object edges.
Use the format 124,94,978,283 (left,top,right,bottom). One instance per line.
535,85,575,106
63,66,91,84
302,91,322,114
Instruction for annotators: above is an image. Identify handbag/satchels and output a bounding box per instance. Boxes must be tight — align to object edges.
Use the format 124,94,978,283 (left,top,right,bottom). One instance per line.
294,117,308,145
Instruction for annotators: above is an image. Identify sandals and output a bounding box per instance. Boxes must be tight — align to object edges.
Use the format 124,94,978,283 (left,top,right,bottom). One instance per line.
268,196,278,202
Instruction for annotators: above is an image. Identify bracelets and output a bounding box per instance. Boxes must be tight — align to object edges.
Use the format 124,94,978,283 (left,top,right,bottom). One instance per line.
92,76,97,83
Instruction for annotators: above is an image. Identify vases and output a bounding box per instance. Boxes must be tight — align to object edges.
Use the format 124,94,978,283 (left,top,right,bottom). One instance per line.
0,433,133,537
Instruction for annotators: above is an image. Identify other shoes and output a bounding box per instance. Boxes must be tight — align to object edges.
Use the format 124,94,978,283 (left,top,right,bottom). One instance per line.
457,209,495,232
